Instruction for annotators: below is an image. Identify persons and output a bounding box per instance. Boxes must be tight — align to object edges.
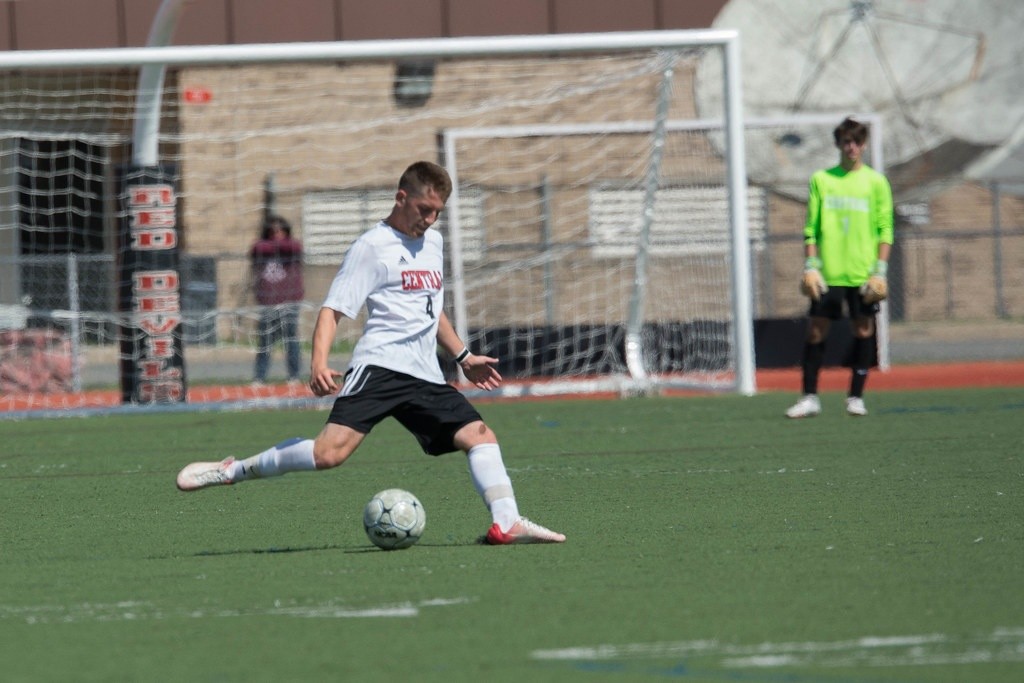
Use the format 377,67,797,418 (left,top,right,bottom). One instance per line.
175,160,566,543
247,214,305,388
785,115,894,418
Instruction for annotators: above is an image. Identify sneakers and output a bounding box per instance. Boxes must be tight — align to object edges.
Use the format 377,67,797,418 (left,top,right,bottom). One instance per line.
176,455,235,492
846,397,869,416
785,395,822,418
487,516,567,543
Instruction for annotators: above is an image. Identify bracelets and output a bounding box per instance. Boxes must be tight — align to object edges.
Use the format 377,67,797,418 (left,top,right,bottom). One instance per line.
455,346,472,364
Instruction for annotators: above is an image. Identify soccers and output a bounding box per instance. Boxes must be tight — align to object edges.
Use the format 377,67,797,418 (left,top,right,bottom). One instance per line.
362,489,427,549
